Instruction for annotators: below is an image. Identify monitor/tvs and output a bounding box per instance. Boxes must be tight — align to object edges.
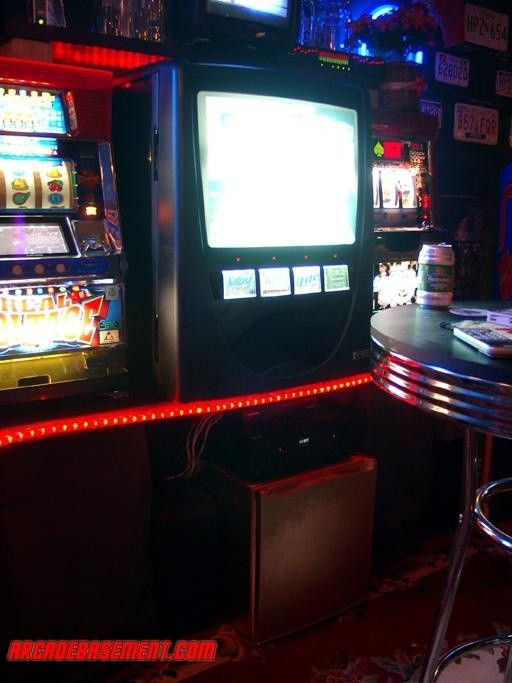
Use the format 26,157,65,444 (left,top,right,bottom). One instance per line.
369,162,420,223
190,86,364,256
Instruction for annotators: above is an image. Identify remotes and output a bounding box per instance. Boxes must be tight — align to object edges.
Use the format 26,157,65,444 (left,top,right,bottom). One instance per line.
453,327,511,358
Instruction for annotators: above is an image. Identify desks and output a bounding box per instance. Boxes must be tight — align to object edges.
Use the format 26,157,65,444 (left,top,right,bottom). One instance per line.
367,297,511,682
